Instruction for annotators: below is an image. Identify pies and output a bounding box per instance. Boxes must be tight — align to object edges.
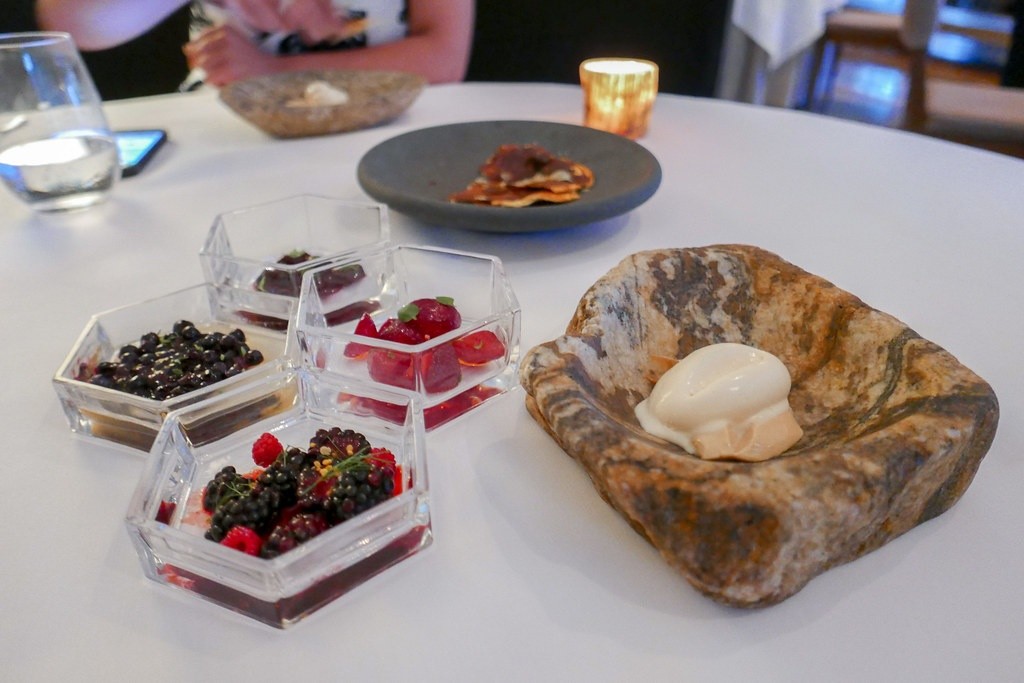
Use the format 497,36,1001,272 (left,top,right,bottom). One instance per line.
447,145,594,208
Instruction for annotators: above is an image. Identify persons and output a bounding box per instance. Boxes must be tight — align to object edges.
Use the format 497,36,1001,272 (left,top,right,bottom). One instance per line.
36,2,473,90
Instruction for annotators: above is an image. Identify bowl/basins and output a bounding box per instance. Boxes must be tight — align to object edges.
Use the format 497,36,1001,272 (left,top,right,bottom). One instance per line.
199,192,392,339
52,281,306,456
294,242,521,433
125,368,432,629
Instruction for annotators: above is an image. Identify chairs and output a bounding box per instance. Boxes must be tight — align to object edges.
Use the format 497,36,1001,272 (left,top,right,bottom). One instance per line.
803,0,1024,160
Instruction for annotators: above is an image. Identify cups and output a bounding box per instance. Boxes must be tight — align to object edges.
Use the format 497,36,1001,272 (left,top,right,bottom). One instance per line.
0,32,120,213
580,57,660,138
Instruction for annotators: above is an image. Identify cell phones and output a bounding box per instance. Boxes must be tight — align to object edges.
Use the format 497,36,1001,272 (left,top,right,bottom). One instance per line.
113,130,166,177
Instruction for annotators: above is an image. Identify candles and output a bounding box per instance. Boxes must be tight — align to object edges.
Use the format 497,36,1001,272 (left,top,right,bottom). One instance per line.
582,56,658,143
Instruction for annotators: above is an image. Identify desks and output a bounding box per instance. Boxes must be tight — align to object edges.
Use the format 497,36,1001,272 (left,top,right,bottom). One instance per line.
0,82,1024,683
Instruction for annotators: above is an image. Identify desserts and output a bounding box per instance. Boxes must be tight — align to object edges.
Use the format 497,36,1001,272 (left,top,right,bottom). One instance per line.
634,343,804,462
284,80,347,106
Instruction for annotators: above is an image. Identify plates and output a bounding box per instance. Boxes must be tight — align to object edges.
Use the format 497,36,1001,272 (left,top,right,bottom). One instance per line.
357,121,663,232
218,68,422,137
